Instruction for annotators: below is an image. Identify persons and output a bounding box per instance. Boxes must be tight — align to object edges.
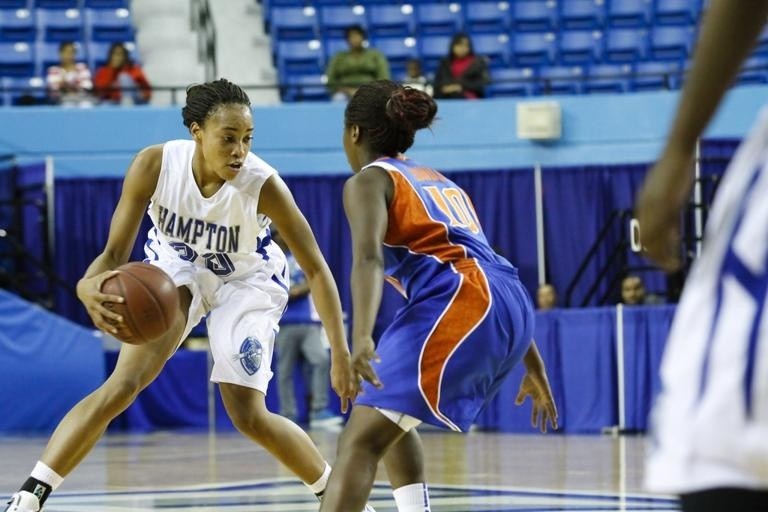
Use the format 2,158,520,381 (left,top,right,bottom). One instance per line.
90,37,152,103
633,2,768,510
269,224,343,427
3,78,376,512
44,38,92,108
620,274,666,304
537,279,558,309
326,25,392,101
319,81,559,512
436,33,489,96
402,59,435,100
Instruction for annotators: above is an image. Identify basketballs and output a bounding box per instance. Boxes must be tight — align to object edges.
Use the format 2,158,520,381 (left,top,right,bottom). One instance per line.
99,263,179,346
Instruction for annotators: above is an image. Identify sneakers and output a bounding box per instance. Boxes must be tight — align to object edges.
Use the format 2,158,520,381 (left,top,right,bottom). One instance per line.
4,490,42,512
